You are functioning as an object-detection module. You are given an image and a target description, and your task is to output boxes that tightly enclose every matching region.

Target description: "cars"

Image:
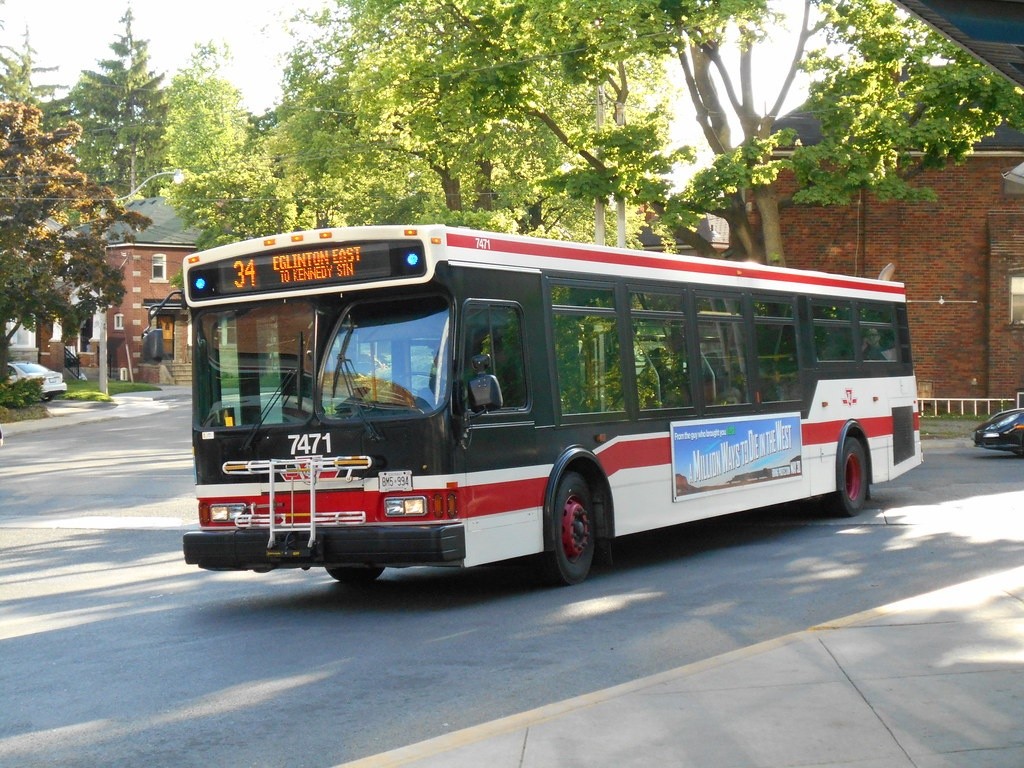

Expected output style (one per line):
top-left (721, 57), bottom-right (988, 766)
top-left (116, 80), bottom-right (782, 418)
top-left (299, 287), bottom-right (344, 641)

top-left (204, 395), bottom-right (313, 426)
top-left (7, 361), bottom-right (67, 401)
top-left (971, 408), bottom-right (1024, 457)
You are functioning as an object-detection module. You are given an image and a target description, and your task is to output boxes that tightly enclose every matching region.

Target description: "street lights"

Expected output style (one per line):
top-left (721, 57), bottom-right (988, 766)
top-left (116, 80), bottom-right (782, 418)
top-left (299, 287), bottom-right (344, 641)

top-left (100, 170), bottom-right (184, 394)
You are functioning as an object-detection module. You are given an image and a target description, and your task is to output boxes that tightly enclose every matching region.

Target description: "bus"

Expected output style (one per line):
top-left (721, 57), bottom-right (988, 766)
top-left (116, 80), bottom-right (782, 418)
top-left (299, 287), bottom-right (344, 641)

top-left (141, 225), bottom-right (924, 585)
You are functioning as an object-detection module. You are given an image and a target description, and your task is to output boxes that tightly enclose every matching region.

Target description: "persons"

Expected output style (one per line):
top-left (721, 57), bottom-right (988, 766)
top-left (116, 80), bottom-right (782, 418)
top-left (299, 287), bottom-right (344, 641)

top-left (862, 329), bottom-right (890, 360)
top-left (639, 349), bottom-right (744, 407)
